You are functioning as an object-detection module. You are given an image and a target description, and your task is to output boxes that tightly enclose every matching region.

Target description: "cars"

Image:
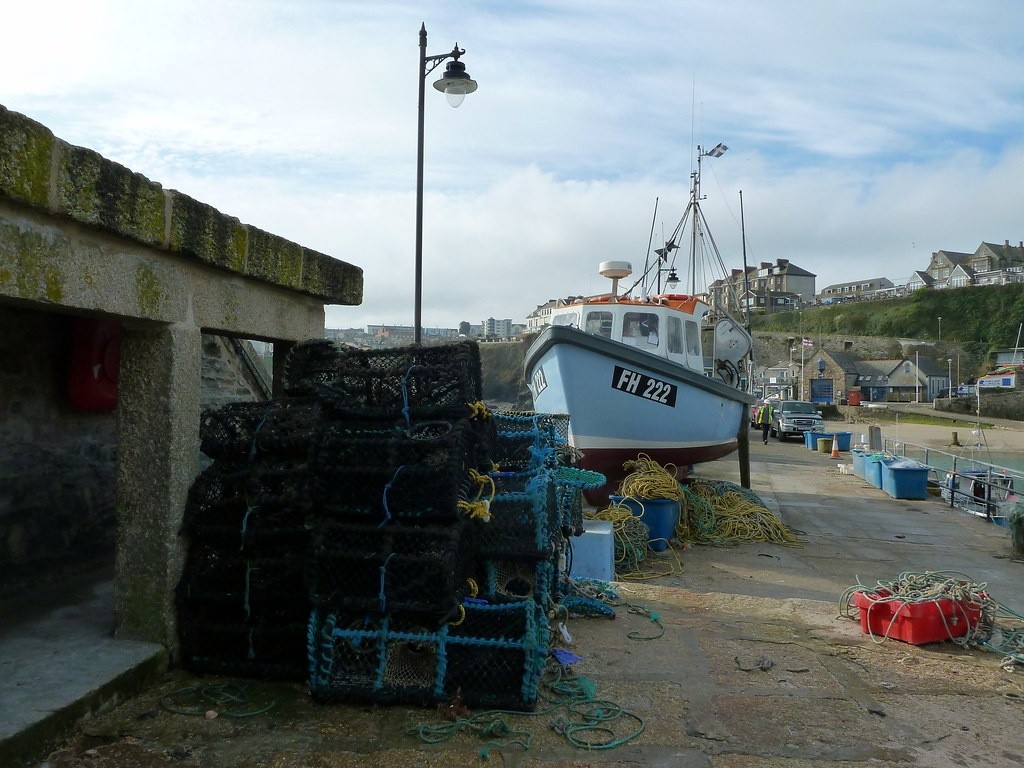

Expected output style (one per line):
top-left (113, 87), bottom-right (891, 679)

top-left (751, 399), bottom-right (781, 430)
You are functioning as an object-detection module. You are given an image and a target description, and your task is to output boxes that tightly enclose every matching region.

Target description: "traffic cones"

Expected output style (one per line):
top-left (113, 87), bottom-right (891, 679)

top-left (828, 433), bottom-right (843, 460)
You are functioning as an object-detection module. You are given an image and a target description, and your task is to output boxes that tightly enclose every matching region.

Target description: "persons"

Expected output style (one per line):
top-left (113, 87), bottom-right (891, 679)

top-left (757, 399), bottom-right (776, 445)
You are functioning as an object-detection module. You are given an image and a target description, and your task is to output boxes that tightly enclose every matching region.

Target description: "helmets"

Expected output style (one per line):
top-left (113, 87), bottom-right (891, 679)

top-left (763, 399), bottom-right (771, 404)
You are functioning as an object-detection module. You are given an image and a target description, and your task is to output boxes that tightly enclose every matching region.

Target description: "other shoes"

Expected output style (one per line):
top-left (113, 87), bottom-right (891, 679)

top-left (765, 440), bottom-right (768, 444)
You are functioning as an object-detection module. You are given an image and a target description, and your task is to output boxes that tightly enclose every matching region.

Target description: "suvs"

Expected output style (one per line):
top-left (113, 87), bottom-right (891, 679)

top-left (769, 400), bottom-right (825, 443)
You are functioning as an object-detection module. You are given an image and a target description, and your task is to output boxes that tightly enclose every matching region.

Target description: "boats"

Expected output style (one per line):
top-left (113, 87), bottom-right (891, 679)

top-left (937, 381), bottom-right (1022, 528)
top-left (519, 72), bottom-right (758, 508)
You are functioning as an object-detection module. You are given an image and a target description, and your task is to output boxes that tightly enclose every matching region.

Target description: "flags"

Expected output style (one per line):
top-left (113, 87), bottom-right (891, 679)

top-left (803, 339), bottom-right (813, 347)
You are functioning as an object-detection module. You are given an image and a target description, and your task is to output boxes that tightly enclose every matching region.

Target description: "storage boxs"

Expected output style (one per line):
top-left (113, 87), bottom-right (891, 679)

top-left (567, 520), bottom-right (615, 584)
top-left (804, 430), bottom-right (851, 452)
top-left (608, 495), bottom-right (678, 551)
top-left (855, 585), bottom-right (990, 647)
top-left (854, 449), bottom-right (933, 499)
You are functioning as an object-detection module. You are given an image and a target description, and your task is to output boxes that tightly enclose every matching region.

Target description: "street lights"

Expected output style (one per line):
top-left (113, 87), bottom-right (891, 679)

top-left (407, 21), bottom-right (480, 386)
top-left (937, 316), bottom-right (943, 342)
top-left (946, 358), bottom-right (955, 404)
top-left (798, 312), bottom-right (803, 333)
